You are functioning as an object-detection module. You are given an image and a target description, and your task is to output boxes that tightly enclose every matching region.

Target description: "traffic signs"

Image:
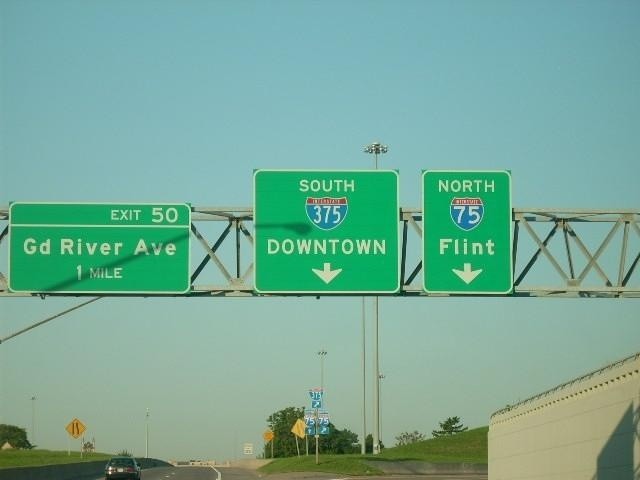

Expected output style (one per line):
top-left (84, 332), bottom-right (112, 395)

top-left (9, 202), bottom-right (189, 293)
top-left (318, 416), bottom-right (330, 426)
top-left (304, 416), bottom-right (315, 426)
top-left (422, 170), bottom-right (512, 293)
top-left (310, 387), bottom-right (325, 400)
top-left (251, 167), bottom-right (401, 293)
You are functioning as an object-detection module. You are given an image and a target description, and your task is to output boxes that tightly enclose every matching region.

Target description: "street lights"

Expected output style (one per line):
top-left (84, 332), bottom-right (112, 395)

top-left (363, 140), bottom-right (391, 454)
top-left (30, 395), bottom-right (37, 450)
top-left (317, 349), bottom-right (328, 386)
top-left (144, 408), bottom-right (152, 457)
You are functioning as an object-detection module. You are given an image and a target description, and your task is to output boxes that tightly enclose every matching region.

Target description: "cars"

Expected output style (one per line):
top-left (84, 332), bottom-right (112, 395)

top-left (103, 454), bottom-right (140, 480)
